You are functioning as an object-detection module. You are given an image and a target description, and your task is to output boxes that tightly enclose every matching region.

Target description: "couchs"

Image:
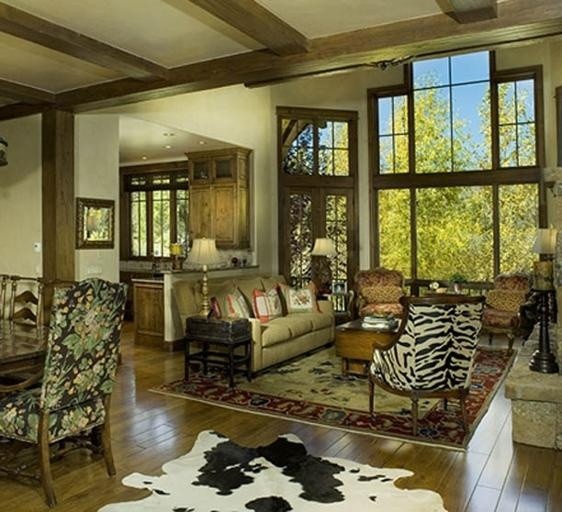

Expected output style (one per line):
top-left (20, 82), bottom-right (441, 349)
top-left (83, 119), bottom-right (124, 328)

top-left (355, 267), bottom-right (407, 318)
top-left (172, 274), bottom-right (335, 372)
top-left (483, 273), bottom-right (530, 351)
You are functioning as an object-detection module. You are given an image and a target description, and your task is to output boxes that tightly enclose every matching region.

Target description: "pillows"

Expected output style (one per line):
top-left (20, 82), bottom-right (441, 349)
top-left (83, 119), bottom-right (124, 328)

top-left (233, 279), bottom-right (263, 317)
top-left (193, 279), bottom-right (234, 311)
top-left (279, 280), bottom-right (320, 313)
top-left (252, 285), bottom-right (282, 323)
top-left (224, 287), bottom-right (250, 318)
top-left (261, 275), bottom-right (287, 313)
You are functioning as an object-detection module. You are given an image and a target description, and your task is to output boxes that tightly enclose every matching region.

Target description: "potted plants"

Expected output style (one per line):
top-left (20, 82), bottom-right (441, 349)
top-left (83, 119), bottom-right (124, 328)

top-left (448, 272), bottom-right (470, 294)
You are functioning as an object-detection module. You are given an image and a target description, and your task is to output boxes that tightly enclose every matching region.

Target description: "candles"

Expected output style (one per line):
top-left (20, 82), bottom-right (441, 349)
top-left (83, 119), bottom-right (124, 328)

top-left (532, 260), bottom-right (553, 290)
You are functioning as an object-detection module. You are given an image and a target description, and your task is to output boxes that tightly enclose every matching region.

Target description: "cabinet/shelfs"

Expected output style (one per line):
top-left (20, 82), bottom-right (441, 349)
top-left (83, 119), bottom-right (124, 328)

top-left (184, 148), bottom-right (253, 249)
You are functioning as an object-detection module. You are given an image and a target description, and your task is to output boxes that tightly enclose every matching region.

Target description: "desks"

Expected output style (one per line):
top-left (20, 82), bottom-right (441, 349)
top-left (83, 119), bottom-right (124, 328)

top-left (0, 317), bottom-right (49, 393)
top-left (335, 316), bottom-right (402, 377)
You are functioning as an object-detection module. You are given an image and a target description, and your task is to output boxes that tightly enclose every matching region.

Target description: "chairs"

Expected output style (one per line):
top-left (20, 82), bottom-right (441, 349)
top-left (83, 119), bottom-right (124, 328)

top-left (52, 278), bottom-right (79, 304)
top-left (369, 296), bottom-right (486, 437)
top-left (0, 274), bottom-right (9, 319)
top-left (0, 278), bottom-right (129, 508)
top-left (9, 277), bottom-right (44, 326)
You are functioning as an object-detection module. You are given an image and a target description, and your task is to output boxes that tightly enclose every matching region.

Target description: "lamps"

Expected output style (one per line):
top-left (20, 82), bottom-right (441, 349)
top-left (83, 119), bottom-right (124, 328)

top-left (531, 229), bottom-right (558, 324)
top-left (310, 238), bottom-right (337, 293)
top-left (169, 243), bottom-right (183, 270)
top-left (183, 237), bottom-right (227, 316)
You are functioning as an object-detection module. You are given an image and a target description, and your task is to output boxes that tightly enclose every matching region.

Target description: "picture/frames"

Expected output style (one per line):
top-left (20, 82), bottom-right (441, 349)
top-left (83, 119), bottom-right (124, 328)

top-left (75, 197), bottom-right (115, 249)
top-left (332, 279), bottom-right (348, 294)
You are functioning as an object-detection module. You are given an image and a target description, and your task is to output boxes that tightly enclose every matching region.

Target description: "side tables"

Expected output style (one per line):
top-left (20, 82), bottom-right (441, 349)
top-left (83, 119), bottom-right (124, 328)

top-left (317, 290), bottom-right (354, 321)
top-left (184, 316), bottom-right (252, 387)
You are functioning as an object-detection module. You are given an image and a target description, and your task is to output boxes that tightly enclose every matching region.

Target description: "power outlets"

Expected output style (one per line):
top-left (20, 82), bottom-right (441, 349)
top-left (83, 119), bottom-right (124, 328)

top-left (33, 242), bottom-right (41, 254)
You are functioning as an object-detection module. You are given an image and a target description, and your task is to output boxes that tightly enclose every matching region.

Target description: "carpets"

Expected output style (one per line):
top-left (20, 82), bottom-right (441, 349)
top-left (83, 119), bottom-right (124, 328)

top-left (147, 342), bottom-right (518, 452)
top-left (97, 430), bottom-right (449, 512)
